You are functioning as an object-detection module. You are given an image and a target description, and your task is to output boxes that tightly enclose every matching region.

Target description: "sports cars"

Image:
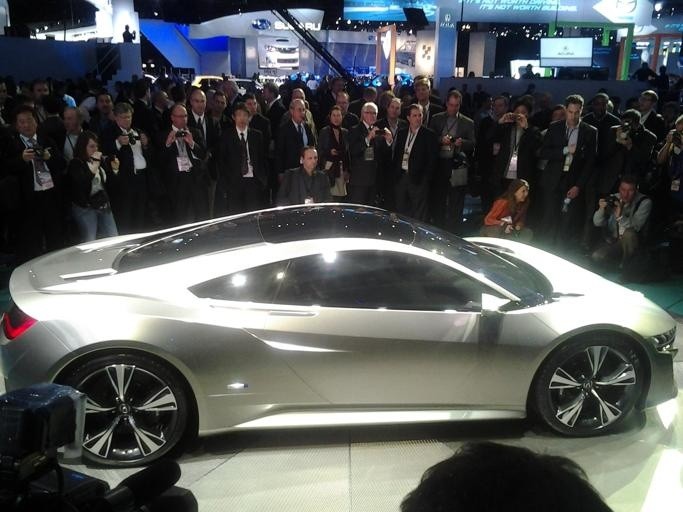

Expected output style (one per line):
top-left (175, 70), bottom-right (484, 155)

top-left (0, 202), bottom-right (679, 467)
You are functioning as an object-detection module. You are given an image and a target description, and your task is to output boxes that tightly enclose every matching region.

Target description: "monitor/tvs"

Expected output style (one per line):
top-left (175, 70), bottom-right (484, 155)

top-left (540, 37), bottom-right (593, 67)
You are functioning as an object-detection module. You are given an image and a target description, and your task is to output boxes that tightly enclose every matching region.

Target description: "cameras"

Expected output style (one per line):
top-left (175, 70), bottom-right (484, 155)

top-left (671, 131), bottom-right (683, 146)
top-left (511, 114), bottom-right (521, 121)
top-left (449, 135), bottom-right (461, 144)
top-left (620, 121), bottom-right (633, 139)
top-left (375, 129), bottom-right (385, 135)
top-left (175, 131), bottom-right (188, 137)
top-left (126, 132), bottom-right (140, 144)
top-left (28, 145), bottom-right (44, 158)
top-left (101, 154), bottom-right (116, 165)
top-left (605, 196), bottom-right (619, 208)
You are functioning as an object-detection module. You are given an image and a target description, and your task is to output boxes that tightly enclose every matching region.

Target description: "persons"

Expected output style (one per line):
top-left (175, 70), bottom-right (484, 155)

top-left (1, 62), bottom-right (683, 275)
top-left (399, 439), bottom-right (617, 511)
top-left (122, 24), bottom-right (135, 43)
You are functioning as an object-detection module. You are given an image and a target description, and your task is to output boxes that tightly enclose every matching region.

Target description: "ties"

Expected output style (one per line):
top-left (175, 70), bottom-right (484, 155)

top-left (240, 133), bottom-right (248, 175)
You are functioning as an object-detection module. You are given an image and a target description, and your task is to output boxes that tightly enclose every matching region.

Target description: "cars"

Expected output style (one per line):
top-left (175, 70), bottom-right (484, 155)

top-left (395, 41), bottom-right (416, 68)
top-left (228, 78), bottom-right (262, 95)
top-left (264, 39), bottom-right (299, 71)
top-left (190, 75), bottom-right (223, 90)
